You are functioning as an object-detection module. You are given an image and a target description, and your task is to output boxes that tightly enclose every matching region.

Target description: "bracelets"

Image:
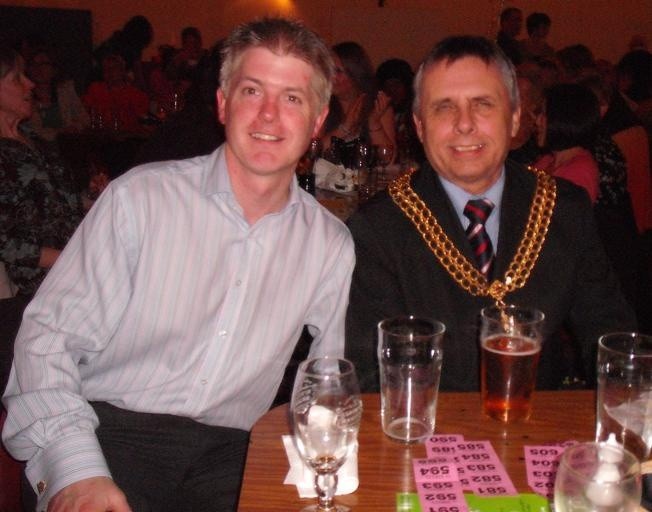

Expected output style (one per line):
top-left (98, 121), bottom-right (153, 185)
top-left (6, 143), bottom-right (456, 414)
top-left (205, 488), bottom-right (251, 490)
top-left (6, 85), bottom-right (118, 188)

top-left (366, 124), bottom-right (386, 132)
top-left (338, 123), bottom-right (352, 136)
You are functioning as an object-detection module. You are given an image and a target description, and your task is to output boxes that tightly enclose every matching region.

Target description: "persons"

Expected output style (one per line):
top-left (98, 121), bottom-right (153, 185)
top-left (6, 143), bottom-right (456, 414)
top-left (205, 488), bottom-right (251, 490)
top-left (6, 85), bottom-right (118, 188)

top-left (24, 45), bottom-right (90, 142)
top-left (527, 82), bottom-right (606, 177)
top-left (535, 62), bottom-right (558, 90)
top-left (85, 54), bottom-right (165, 143)
top-left (168, 26), bottom-right (208, 78)
top-left (558, 45), bottom-right (614, 123)
top-left (0, 48), bottom-right (109, 300)
top-left (85, 15), bottom-right (154, 92)
top-left (0, 8), bottom-right (357, 512)
top-left (523, 13), bottom-right (561, 64)
top-left (284, 35), bottom-right (646, 392)
top-left (136, 55), bottom-right (225, 163)
top-left (293, 44), bottom-right (395, 177)
top-left (154, 62), bottom-right (199, 123)
top-left (602, 50), bottom-right (652, 136)
top-left (510, 75), bottom-right (539, 167)
top-left (493, 7), bottom-right (540, 74)
top-left (369, 57), bottom-right (423, 164)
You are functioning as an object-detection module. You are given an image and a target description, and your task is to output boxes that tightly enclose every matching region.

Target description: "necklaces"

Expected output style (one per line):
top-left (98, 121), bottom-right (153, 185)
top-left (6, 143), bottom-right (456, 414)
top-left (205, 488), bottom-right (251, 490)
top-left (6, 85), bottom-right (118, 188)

top-left (388, 159), bottom-right (557, 337)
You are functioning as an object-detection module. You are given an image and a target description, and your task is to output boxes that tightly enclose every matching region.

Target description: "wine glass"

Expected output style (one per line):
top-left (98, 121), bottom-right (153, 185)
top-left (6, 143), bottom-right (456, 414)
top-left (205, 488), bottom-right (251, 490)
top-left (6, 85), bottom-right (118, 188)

top-left (555, 442), bottom-right (643, 512)
top-left (290, 356), bottom-right (363, 512)
top-left (375, 144), bottom-right (394, 187)
top-left (352, 144), bottom-right (371, 192)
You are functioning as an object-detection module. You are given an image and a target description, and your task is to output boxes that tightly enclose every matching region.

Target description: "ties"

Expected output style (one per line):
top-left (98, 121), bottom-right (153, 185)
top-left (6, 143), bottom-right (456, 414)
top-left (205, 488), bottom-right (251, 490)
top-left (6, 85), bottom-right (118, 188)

top-left (464, 198), bottom-right (495, 280)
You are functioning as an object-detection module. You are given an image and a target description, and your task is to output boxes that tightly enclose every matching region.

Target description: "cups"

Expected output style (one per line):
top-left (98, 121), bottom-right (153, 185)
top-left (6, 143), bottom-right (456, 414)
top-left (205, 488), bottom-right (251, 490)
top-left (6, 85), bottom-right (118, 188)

top-left (596, 333), bottom-right (652, 464)
top-left (481, 305), bottom-right (546, 426)
top-left (300, 139), bottom-right (319, 195)
top-left (379, 315), bottom-right (445, 444)
